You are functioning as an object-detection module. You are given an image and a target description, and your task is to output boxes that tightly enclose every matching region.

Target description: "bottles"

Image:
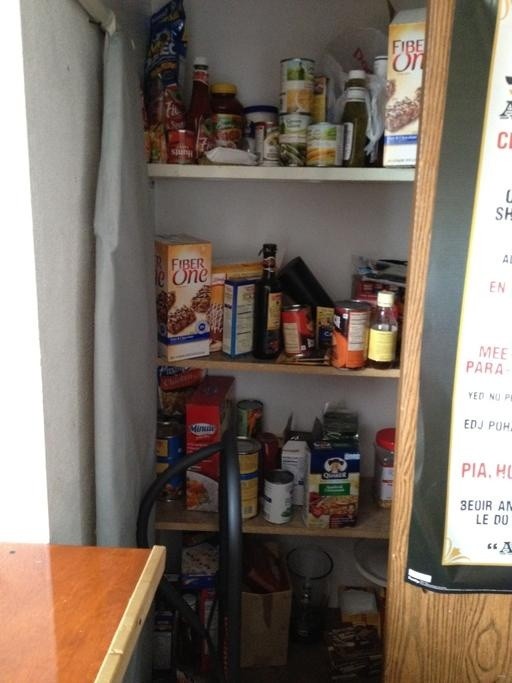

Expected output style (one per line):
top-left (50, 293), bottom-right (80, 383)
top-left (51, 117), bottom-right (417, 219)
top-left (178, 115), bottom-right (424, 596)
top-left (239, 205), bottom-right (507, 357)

top-left (152, 610), bottom-right (174, 676)
top-left (185, 56), bottom-right (212, 162)
top-left (213, 81), bottom-right (245, 157)
top-left (371, 427), bottom-right (396, 510)
top-left (367, 289), bottom-right (403, 368)
top-left (368, 52), bottom-right (387, 165)
top-left (255, 241), bottom-right (282, 358)
top-left (341, 68), bottom-right (370, 165)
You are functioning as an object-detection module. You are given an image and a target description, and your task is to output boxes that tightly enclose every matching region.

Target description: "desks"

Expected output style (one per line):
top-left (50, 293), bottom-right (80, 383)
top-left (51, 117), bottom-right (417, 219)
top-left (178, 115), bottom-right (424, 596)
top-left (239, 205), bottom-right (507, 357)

top-left (1, 540), bottom-right (168, 682)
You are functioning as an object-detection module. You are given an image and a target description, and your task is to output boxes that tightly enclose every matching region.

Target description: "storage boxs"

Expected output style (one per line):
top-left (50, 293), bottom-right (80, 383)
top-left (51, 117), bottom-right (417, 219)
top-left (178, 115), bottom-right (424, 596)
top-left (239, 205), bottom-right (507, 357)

top-left (238, 550), bottom-right (293, 668)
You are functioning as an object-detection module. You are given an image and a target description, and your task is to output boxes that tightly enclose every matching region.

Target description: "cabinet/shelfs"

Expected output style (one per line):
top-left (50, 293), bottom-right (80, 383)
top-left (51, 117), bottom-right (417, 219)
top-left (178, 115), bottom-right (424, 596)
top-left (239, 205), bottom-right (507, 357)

top-left (144, 157), bottom-right (420, 542)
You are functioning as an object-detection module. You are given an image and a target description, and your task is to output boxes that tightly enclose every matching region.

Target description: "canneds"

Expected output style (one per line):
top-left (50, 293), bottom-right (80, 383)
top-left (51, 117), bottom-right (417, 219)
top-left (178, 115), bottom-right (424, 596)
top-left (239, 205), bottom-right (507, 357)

top-left (374, 428), bottom-right (395, 511)
top-left (280, 303), bottom-right (316, 359)
top-left (236, 399), bottom-right (294, 525)
top-left (154, 421), bottom-right (186, 503)
top-left (242, 57), bottom-right (345, 166)
top-left (166, 129), bottom-right (196, 165)
top-left (329, 299), bottom-right (372, 371)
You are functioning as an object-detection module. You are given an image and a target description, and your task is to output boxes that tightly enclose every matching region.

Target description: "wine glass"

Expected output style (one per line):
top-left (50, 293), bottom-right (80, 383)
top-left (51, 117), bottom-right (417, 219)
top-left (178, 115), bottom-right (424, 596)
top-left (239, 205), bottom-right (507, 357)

top-left (285, 545), bottom-right (336, 642)
